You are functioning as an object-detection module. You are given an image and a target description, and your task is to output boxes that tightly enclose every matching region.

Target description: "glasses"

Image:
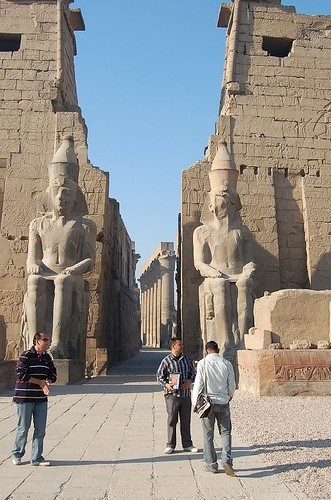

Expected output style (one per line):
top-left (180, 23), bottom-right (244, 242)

top-left (39, 338), bottom-right (49, 342)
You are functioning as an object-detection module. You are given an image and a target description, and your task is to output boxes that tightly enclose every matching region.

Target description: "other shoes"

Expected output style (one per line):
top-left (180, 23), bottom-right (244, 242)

top-left (31, 461), bottom-right (51, 466)
top-left (183, 445), bottom-right (199, 453)
top-left (205, 464), bottom-right (219, 474)
top-left (220, 462), bottom-right (234, 476)
top-left (13, 455), bottom-right (22, 465)
top-left (163, 447), bottom-right (175, 454)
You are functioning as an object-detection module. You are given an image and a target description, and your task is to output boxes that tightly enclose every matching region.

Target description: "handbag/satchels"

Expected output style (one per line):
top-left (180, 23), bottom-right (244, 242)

top-left (193, 393), bottom-right (212, 419)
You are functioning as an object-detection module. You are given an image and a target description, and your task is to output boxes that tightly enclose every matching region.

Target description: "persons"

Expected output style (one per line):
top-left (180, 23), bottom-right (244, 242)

top-left (156, 338), bottom-right (199, 454)
top-left (191, 341), bottom-right (236, 477)
top-left (26, 134), bottom-right (96, 358)
top-left (193, 138), bottom-right (257, 348)
top-left (11, 331), bottom-right (57, 466)
